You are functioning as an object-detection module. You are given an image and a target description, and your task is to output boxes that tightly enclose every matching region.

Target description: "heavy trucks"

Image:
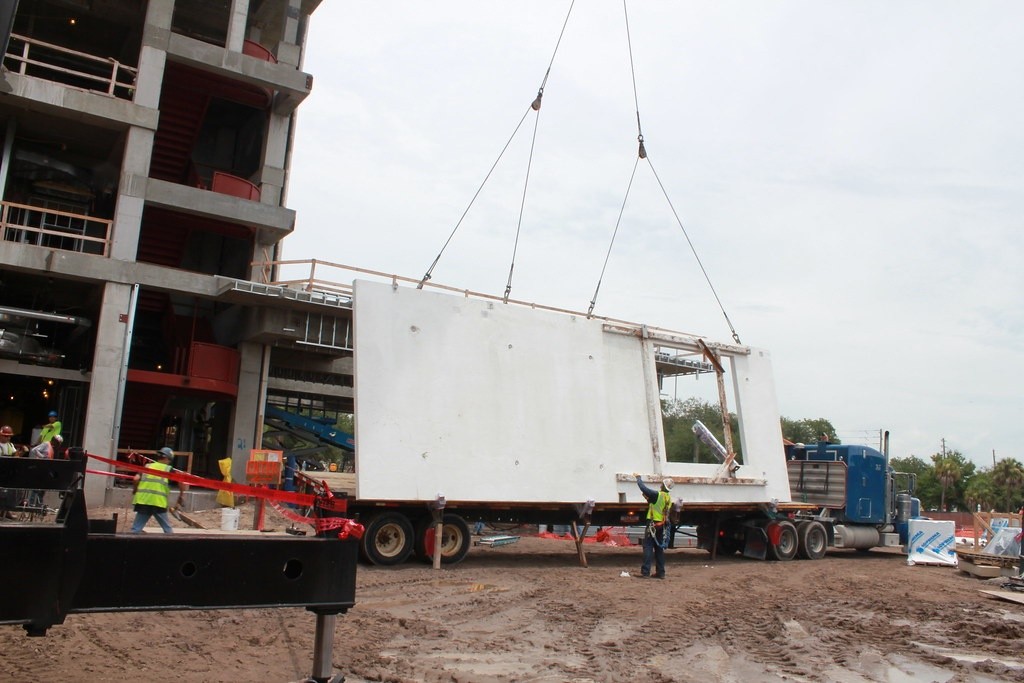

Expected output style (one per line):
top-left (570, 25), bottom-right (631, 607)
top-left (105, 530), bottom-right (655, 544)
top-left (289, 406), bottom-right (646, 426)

top-left (267, 247), bottom-right (930, 569)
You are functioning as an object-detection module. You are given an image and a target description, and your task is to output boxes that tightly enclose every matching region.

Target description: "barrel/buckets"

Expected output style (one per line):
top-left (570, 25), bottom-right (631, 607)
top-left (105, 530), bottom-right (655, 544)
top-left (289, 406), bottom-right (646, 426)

top-left (219, 507), bottom-right (241, 531)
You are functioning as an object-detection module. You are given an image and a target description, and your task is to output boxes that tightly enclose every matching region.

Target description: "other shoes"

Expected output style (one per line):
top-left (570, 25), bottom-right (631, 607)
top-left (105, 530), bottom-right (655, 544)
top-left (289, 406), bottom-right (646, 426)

top-left (651, 574), bottom-right (666, 579)
top-left (633, 574), bottom-right (650, 578)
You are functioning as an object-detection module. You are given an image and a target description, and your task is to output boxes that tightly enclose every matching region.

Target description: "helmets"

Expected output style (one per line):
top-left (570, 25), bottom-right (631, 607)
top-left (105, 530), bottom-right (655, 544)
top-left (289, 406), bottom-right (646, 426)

top-left (0, 425), bottom-right (14, 435)
top-left (663, 478), bottom-right (674, 491)
top-left (48, 410), bottom-right (58, 416)
top-left (157, 446), bottom-right (174, 461)
top-left (53, 435), bottom-right (63, 444)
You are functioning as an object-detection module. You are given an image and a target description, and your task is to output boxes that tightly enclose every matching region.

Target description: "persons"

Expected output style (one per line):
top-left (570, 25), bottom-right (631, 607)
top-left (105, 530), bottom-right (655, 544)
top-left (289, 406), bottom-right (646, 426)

top-left (0, 426), bottom-right (27, 519)
top-left (635, 473), bottom-right (675, 579)
top-left (27, 434), bottom-right (64, 515)
top-left (38, 412), bottom-right (61, 444)
top-left (472, 521), bottom-right (485, 536)
top-left (130, 447), bottom-right (175, 533)
